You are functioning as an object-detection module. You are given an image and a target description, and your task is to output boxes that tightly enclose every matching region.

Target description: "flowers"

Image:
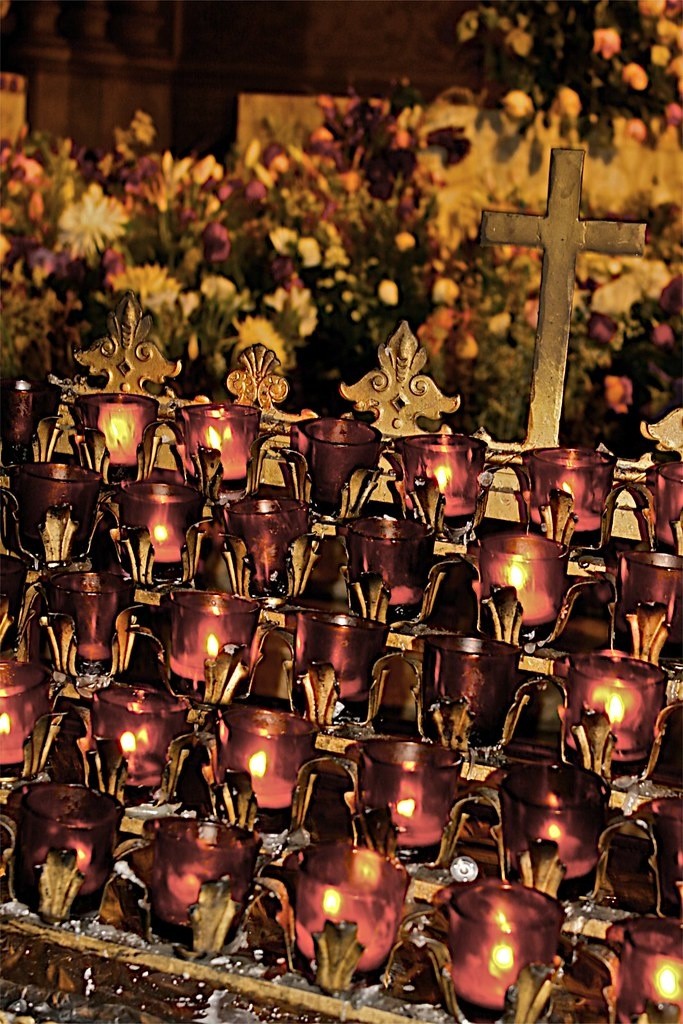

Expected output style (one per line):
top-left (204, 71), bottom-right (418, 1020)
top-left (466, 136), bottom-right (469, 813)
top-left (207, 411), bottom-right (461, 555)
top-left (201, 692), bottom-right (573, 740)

top-left (0, 0), bottom-right (683, 461)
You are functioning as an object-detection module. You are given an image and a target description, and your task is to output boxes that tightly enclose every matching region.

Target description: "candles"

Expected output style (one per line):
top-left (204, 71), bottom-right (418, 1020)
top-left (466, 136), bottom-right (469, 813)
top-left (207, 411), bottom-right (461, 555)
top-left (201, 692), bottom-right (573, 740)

top-left (604, 918), bottom-right (683, 1024)
top-left (639, 797), bottom-right (683, 918)
top-left (5, 782), bottom-right (125, 917)
top-left (88, 685), bottom-right (190, 795)
top-left (0, 376), bottom-right (683, 626)
top-left (430, 883), bottom-right (565, 1010)
top-left (346, 737), bottom-right (461, 849)
top-left (423, 633), bottom-right (522, 746)
top-left (284, 847), bottom-right (411, 981)
top-left (141, 818), bottom-right (264, 947)
top-left (41, 571), bottom-right (135, 672)
top-left (156, 588), bottom-right (260, 691)
top-left (219, 703), bottom-right (318, 810)
top-left (552, 653), bottom-right (668, 775)
top-left (0, 660), bottom-right (52, 774)
top-left (485, 761), bottom-right (613, 888)
top-left (292, 609), bottom-right (389, 718)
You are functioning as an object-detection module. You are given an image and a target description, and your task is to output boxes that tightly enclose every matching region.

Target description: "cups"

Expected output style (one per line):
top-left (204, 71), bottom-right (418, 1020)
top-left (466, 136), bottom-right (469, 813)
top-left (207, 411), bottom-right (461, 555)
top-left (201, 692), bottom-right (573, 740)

top-left (0, 381), bottom-right (683, 1024)
